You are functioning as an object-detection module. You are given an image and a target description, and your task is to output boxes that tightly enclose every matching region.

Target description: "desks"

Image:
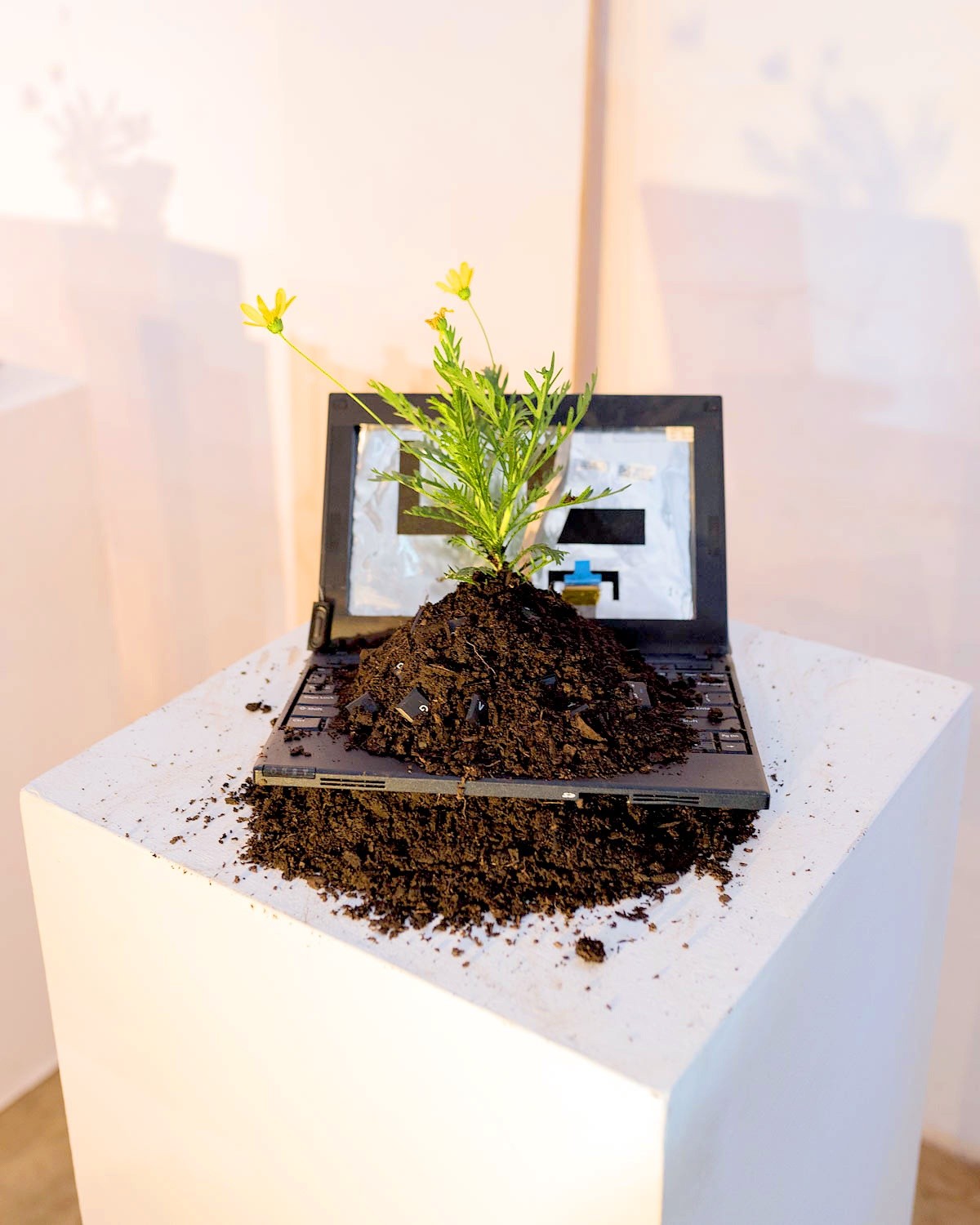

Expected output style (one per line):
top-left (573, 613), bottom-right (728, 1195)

top-left (11, 619), bottom-right (976, 1225)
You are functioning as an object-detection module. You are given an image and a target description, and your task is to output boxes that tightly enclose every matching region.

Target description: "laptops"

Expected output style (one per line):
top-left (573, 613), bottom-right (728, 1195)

top-left (255, 391), bottom-right (771, 812)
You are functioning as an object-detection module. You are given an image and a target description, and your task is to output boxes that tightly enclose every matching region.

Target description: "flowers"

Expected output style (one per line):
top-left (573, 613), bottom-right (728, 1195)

top-left (234, 254), bottom-right (637, 590)
